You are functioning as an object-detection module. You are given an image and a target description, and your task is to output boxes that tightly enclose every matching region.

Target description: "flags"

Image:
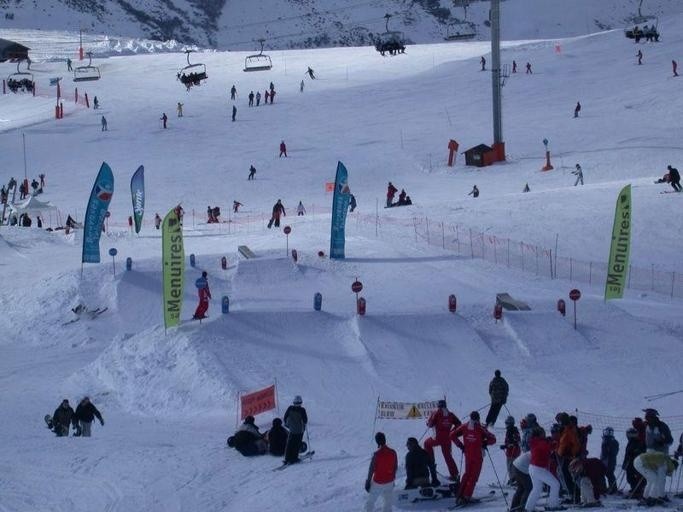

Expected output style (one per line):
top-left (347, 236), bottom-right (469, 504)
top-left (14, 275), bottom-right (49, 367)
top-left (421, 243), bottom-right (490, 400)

top-left (132, 166), bottom-right (144, 232)
top-left (330, 162), bottom-right (348, 258)
top-left (83, 161), bottom-right (115, 262)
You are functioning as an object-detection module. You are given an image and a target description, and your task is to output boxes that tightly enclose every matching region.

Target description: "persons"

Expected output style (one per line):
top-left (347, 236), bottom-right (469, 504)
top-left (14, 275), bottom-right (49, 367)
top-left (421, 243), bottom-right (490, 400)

top-left (160, 72), bottom-right (200, 128)
top-left (485, 370), bottom-right (509, 427)
top-left (8, 78), bottom-right (33, 92)
top-left (449, 411), bottom-right (497, 505)
top-left (386, 181), bottom-right (411, 207)
top-left (75, 396), bottom-right (104, 437)
top-left (66, 58), bottom-right (72, 71)
top-left (279, 140), bottom-right (287, 157)
top-left (193, 271), bottom-right (212, 318)
top-left (129, 213), bottom-right (162, 229)
top-left (405, 400), bottom-right (462, 490)
top-left (364, 432), bottom-right (398, 511)
top-left (349, 194), bottom-right (356, 211)
top-left (623, 409), bottom-right (683, 506)
top-left (227, 396), bottom-right (308, 464)
top-left (53, 399), bottom-right (75, 437)
top-left (2, 174), bottom-right (76, 231)
top-left (94, 96), bottom-right (107, 130)
top-left (248, 164), bottom-right (255, 179)
top-left (207, 201), bottom-right (243, 223)
top-left (267, 199), bottom-right (305, 228)
top-left (231, 66), bottom-right (315, 121)
top-left (500, 412), bottom-right (619, 512)
top-left (468, 24), bottom-right (683, 197)
top-left (378, 38), bottom-right (405, 56)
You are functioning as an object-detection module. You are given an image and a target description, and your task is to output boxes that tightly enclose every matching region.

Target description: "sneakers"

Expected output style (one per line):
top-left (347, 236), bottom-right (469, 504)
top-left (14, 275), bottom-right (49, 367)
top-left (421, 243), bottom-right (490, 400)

top-left (582, 501), bottom-right (601, 508)
top-left (544, 497), bottom-right (601, 511)
top-left (607, 487), bottom-right (640, 499)
top-left (456, 496), bottom-right (476, 504)
top-left (640, 496), bottom-right (670, 506)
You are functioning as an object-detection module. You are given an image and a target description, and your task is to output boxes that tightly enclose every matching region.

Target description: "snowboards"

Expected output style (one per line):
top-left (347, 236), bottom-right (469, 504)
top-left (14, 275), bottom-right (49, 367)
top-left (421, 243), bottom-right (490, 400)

top-left (393, 486), bottom-right (435, 501)
top-left (45, 414), bottom-right (69, 437)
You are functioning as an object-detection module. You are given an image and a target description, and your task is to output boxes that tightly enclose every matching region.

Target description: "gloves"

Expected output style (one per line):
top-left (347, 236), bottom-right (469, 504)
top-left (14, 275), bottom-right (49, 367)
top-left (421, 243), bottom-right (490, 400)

top-left (500, 445), bottom-right (506, 449)
top-left (365, 480), bottom-right (371, 492)
top-left (460, 446), bottom-right (464, 451)
top-left (482, 444), bottom-right (488, 449)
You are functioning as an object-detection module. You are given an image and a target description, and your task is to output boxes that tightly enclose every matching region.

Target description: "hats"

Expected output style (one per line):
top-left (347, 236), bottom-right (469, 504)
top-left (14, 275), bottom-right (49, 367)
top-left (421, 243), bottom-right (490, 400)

top-left (439, 400), bottom-right (446, 407)
top-left (407, 438), bottom-right (418, 446)
top-left (293, 395), bottom-right (302, 404)
top-left (603, 427), bottom-right (614, 437)
top-left (202, 272), bottom-right (207, 277)
top-left (246, 416), bottom-right (254, 423)
top-left (273, 418), bottom-right (282, 426)
top-left (375, 432), bottom-right (385, 444)
top-left (505, 414), bottom-right (536, 429)
top-left (471, 411), bottom-right (480, 420)
top-left (551, 423), bottom-right (562, 433)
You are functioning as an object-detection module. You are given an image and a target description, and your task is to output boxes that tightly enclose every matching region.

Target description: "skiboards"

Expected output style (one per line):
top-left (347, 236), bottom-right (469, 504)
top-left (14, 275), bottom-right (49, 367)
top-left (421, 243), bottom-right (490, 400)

top-left (438, 491), bottom-right (508, 512)
top-left (272, 449), bottom-right (315, 473)
